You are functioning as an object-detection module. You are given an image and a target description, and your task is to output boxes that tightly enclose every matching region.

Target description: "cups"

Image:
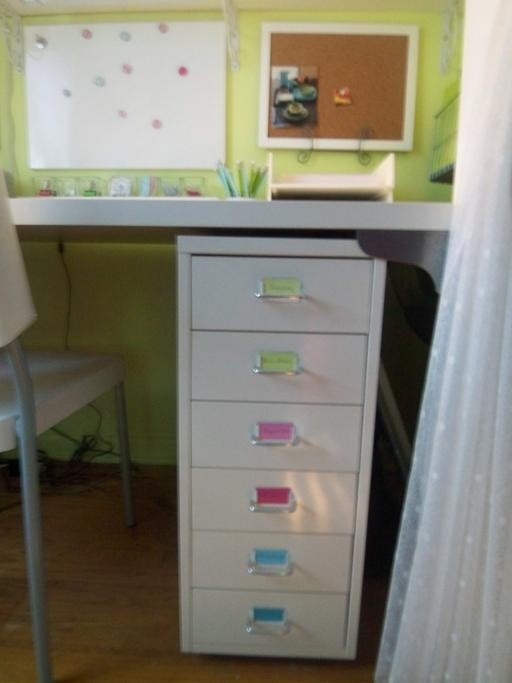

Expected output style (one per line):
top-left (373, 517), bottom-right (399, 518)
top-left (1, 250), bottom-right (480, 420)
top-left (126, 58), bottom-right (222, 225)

top-left (299, 86), bottom-right (315, 97)
top-left (287, 103), bottom-right (304, 116)
top-left (31, 174), bottom-right (205, 197)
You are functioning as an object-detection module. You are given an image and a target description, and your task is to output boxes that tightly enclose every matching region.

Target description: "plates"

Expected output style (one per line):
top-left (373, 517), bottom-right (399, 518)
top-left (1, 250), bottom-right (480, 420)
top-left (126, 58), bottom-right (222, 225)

top-left (293, 87), bottom-right (318, 101)
top-left (281, 107), bottom-right (309, 122)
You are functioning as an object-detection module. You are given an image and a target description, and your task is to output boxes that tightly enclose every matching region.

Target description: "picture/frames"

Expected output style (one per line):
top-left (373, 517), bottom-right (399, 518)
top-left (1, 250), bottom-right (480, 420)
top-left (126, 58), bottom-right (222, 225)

top-left (258, 21), bottom-right (419, 150)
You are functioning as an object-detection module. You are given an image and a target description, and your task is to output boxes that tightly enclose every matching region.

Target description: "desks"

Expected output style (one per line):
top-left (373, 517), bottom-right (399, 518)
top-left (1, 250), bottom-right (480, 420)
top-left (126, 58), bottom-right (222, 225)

top-left (9, 194), bottom-right (454, 475)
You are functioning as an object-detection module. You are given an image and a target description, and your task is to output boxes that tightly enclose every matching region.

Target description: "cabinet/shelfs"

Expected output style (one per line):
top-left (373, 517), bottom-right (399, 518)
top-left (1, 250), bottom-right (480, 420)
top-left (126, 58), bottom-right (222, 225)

top-left (175, 236), bottom-right (395, 664)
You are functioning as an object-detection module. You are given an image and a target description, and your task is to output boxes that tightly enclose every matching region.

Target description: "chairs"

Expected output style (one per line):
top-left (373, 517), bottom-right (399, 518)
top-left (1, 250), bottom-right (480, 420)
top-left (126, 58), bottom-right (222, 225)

top-left (0, 164), bottom-right (150, 683)
